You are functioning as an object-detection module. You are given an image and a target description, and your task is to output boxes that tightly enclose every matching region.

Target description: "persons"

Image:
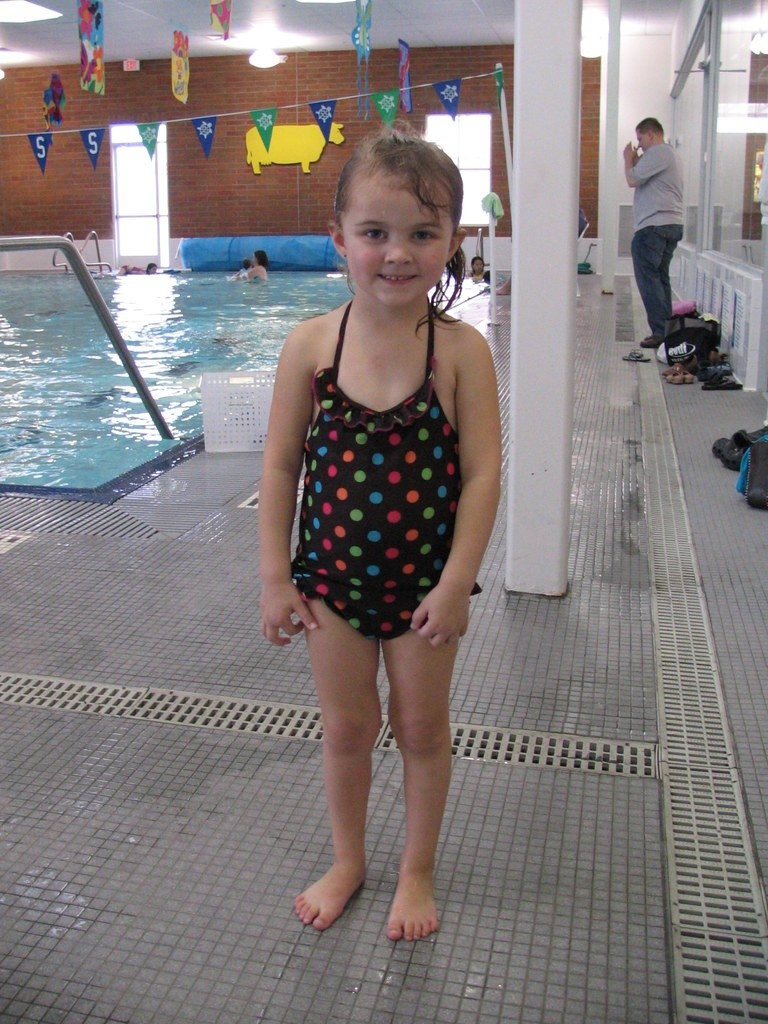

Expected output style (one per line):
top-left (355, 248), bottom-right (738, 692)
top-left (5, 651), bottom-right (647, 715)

top-left (465, 257), bottom-right (511, 296)
top-left (260, 120), bottom-right (501, 939)
top-left (622, 118), bottom-right (686, 352)
top-left (117, 262), bottom-right (158, 277)
top-left (231, 251), bottom-right (269, 285)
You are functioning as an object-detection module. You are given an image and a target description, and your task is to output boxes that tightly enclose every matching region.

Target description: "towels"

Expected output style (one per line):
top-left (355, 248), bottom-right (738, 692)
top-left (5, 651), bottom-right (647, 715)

top-left (481, 192), bottom-right (504, 220)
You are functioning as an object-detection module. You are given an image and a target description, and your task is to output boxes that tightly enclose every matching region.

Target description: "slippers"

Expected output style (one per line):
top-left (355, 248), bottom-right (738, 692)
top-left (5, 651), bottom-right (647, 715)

top-left (622, 349), bottom-right (651, 362)
top-left (665, 371), bottom-right (695, 385)
top-left (700, 373), bottom-right (743, 390)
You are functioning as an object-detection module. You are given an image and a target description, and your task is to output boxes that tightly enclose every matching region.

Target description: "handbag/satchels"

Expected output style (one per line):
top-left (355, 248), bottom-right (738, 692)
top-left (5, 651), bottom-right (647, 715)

top-left (664, 314), bottom-right (719, 365)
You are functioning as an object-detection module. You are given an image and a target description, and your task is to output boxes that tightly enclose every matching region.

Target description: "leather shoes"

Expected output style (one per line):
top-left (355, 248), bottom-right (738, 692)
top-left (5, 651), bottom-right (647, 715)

top-left (640, 336), bottom-right (665, 348)
